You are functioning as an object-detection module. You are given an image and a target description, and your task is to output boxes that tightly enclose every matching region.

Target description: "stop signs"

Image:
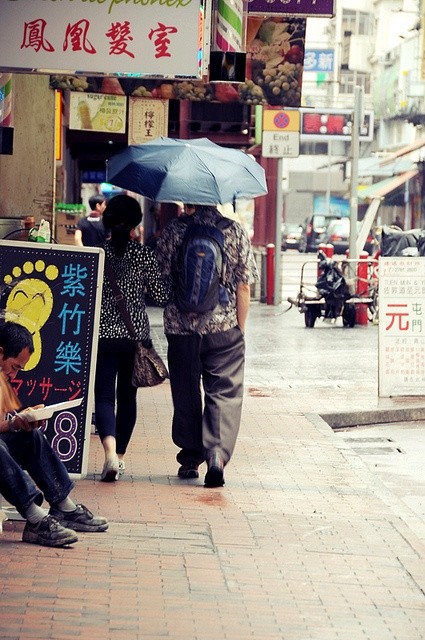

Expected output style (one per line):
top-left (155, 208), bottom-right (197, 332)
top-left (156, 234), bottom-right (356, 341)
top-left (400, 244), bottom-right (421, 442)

top-left (261, 108), bottom-right (301, 158)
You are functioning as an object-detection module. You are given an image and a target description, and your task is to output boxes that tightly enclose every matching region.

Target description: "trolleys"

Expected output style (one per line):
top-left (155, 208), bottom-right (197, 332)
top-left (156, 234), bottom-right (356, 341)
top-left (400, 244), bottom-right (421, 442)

top-left (287, 259), bottom-right (379, 328)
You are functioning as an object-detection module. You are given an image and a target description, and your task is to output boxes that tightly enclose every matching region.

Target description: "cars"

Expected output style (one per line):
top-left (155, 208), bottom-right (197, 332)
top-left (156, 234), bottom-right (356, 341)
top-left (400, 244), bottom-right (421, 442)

top-left (324, 219), bottom-right (374, 256)
top-left (281, 223), bottom-right (303, 251)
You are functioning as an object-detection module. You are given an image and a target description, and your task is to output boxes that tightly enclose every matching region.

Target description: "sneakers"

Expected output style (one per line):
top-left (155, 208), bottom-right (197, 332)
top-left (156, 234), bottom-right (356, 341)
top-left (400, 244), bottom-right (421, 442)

top-left (100, 457), bottom-right (120, 482)
top-left (47, 503), bottom-right (108, 532)
top-left (204, 453), bottom-right (224, 488)
top-left (118, 460), bottom-right (126, 472)
top-left (178, 465), bottom-right (200, 479)
top-left (22, 514), bottom-right (78, 547)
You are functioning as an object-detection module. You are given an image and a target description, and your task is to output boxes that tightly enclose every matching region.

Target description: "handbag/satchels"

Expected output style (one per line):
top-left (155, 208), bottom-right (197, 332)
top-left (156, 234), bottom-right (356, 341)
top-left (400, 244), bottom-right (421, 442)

top-left (131, 343), bottom-right (169, 388)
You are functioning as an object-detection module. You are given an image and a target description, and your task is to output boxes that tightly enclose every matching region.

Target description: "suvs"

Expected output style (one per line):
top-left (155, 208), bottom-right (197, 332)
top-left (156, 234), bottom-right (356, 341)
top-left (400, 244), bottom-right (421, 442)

top-left (299, 214), bottom-right (329, 253)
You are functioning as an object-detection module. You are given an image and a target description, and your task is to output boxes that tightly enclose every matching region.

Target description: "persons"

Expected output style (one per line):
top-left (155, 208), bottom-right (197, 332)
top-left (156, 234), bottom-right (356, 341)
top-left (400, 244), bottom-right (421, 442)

top-left (391, 216), bottom-right (404, 230)
top-left (0, 321), bottom-right (109, 546)
top-left (149, 202), bottom-right (258, 488)
top-left (95, 195), bottom-right (173, 481)
top-left (75, 195), bottom-right (108, 248)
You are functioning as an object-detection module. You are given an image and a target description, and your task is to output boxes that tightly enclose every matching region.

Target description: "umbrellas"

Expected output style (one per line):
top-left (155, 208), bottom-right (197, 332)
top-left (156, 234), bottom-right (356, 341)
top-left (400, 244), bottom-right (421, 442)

top-left (104, 138), bottom-right (270, 234)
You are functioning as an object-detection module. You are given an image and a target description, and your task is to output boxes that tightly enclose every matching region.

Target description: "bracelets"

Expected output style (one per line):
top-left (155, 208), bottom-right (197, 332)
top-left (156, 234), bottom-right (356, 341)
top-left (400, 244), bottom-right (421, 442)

top-left (8, 414), bottom-right (22, 433)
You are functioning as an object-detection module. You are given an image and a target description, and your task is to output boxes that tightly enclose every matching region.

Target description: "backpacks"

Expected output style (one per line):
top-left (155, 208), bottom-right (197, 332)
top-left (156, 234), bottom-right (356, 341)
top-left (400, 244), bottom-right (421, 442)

top-left (169, 214), bottom-right (234, 315)
